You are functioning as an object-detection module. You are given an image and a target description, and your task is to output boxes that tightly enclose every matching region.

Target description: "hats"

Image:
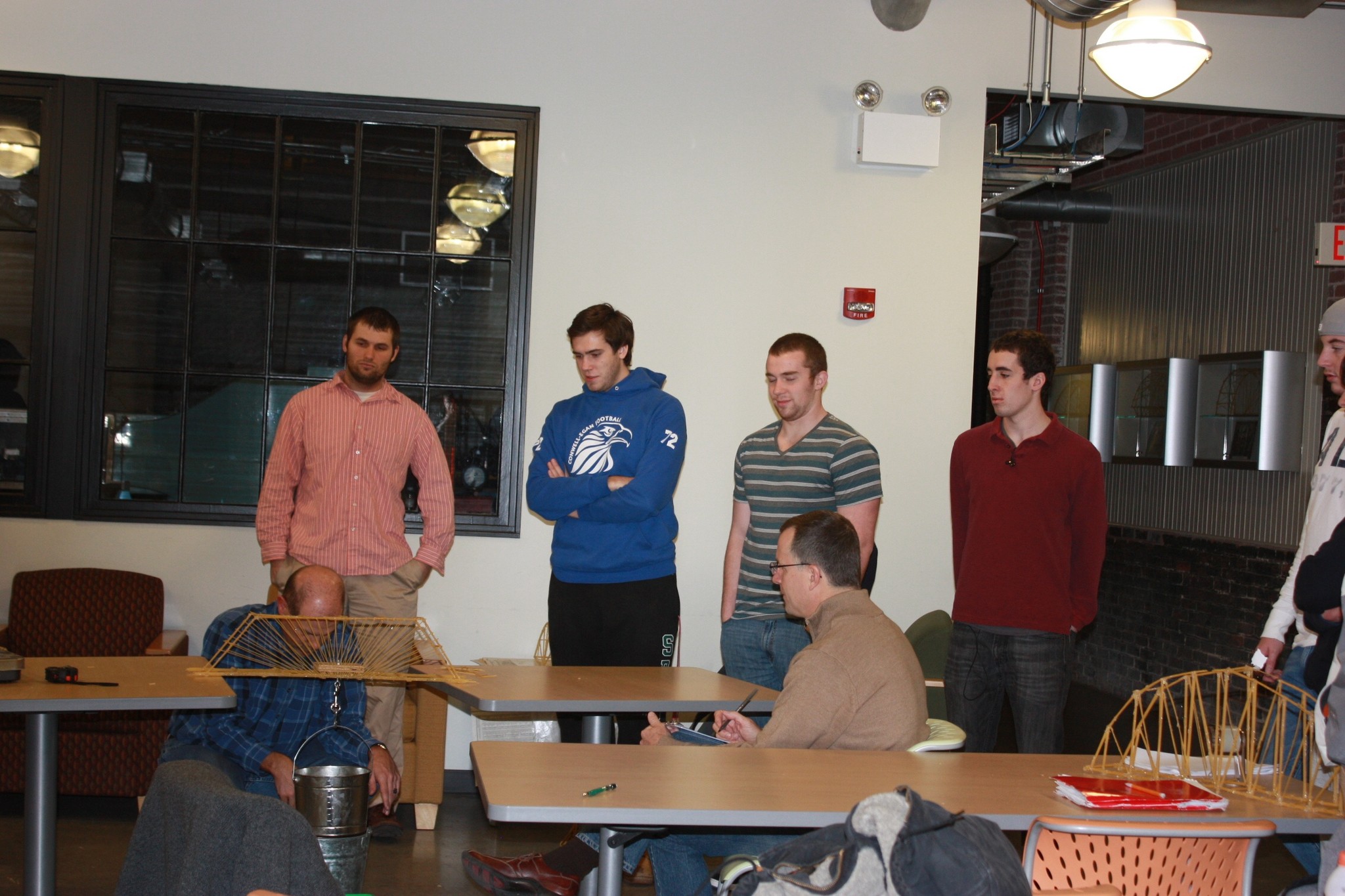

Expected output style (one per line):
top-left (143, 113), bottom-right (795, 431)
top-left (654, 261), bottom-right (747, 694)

top-left (1319, 296), bottom-right (1345, 336)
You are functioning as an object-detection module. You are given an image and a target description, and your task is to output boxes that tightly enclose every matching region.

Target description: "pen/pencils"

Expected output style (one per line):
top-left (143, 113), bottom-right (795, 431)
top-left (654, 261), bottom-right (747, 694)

top-left (583, 783), bottom-right (617, 796)
top-left (1126, 782), bottom-right (1166, 798)
top-left (718, 688), bottom-right (759, 732)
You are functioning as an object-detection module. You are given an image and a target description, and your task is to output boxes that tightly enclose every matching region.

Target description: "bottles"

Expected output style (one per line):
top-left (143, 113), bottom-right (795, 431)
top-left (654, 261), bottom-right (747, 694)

top-left (1324, 851), bottom-right (1345, 896)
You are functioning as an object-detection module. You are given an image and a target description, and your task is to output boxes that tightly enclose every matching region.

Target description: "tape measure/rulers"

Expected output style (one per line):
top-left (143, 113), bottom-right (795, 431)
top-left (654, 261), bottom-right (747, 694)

top-left (45, 665), bottom-right (78, 684)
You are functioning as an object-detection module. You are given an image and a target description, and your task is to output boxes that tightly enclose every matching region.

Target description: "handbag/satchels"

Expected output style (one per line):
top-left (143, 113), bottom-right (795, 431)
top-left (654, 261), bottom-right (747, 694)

top-left (713, 782), bottom-right (1031, 896)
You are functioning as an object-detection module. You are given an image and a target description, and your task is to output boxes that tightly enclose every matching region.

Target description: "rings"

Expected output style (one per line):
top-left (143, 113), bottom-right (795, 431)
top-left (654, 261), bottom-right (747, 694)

top-left (393, 789), bottom-right (397, 794)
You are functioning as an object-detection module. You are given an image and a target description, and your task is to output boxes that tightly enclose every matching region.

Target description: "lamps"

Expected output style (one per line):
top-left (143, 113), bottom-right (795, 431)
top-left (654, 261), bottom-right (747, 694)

top-left (435, 130), bottom-right (515, 263)
top-left (1087, 0), bottom-right (1213, 99)
top-left (853, 79), bottom-right (953, 168)
top-left (0, 119), bottom-right (41, 178)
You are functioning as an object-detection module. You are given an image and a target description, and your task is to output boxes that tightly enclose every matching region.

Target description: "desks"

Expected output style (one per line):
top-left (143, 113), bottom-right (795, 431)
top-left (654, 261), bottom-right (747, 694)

top-left (406, 664), bottom-right (783, 745)
top-left (468, 738), bottom-right (1344, 895)
top-left (0, 655), bottom-right (236, 896)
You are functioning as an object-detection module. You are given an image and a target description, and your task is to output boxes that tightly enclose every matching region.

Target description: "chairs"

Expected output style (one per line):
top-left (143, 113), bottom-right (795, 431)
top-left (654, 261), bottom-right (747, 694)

top-left (716, 717), bottom-right (966, 895)
top-left (1022, 813), bottom-right (1277, 894)
top-left (0, 565), bottom-right (189, 813)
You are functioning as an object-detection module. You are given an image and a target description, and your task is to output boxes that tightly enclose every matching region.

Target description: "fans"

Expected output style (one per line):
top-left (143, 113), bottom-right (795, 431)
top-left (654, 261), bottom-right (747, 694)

top-left (868, 0), bottom-right (1327, 106)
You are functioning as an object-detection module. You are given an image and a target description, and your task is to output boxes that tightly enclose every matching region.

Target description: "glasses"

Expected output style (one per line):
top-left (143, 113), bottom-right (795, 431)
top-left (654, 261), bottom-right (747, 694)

top-left (768, 561), bottom-right (812, 574)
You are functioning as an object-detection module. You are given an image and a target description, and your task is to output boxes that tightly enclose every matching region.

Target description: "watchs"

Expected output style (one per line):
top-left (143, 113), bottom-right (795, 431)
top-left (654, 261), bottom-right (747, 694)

top-left (376, 743), bottom-right (386, 749)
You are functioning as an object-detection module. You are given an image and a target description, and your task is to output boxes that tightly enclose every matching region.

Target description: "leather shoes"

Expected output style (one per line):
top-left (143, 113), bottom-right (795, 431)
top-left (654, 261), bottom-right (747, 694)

top-left (370, 809), bottom-right (401, 839)
top-left (460, 849), bottom-right (582, 893)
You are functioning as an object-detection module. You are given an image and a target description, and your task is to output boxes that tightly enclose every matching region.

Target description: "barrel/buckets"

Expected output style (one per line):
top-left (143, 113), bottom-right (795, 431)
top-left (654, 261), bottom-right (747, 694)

top-left (292, 724), bottom-right (374, 837)
top-left (315, 828), bottom-right (372, 894)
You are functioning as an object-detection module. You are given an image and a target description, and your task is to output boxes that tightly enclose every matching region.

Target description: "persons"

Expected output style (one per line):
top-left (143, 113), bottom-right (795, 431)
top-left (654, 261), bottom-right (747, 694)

top-left (157, 565), bottom-right (403, 817)
top-left (949, 332), bottom-right (1109, 754)
top-left (526, 305), bottom-right (687, 886)
top-left (256, 305), bottom-right (457, 840)
top-left (0, 339), bottom-right (28, 483)
top-left (435, 391), bottom-right (488, 497)
top-left (461, 513), bottom-right (929, 896)
top-left (719, 333), bottom-right (883, 729)
top-left (1250, 297), bottom-right (1345, 896)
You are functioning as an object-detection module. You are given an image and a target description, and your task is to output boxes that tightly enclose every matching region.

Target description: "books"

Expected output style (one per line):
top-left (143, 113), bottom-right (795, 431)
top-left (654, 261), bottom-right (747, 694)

top-left (1052, 772), bottom-right (1227, 808)
